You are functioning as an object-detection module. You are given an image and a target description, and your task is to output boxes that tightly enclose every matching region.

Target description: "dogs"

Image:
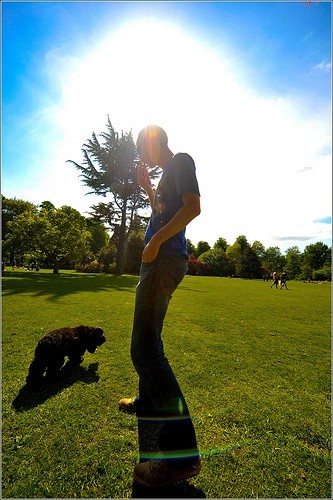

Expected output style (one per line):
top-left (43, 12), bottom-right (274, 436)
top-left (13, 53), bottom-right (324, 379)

top-left (26, 325), bottom-right (105, 380)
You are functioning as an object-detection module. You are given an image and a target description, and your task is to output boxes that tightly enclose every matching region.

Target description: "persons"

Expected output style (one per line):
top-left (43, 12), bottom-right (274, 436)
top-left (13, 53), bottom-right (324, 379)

top-left (116, 125), bottom-right (203, 483)
top-left (280, 270), bottom-right (289, 290)
top-left (271, 270), bottom-right (278, 289)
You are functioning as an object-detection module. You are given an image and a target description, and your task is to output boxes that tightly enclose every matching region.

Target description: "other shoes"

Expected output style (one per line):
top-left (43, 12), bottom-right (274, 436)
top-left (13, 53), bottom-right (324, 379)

top-left (119, 396), bottom-right (137, 410)
top-left (133, 452), bottom-right (201, 487)
